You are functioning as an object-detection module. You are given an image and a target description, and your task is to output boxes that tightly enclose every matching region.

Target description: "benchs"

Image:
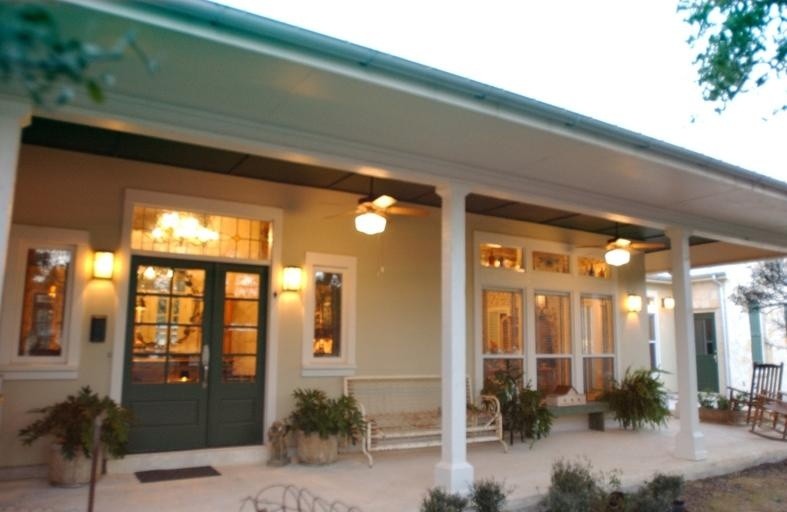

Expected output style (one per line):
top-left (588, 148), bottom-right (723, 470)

top-left (342, 372), bottom-right (510, 469)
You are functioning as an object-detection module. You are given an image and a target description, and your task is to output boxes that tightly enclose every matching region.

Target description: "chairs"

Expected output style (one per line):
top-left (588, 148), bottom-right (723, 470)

top-left (725, 361), bottom-right (786, 441)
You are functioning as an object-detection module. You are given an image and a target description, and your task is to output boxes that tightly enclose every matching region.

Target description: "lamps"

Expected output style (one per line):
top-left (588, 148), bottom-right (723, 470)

top-left (353, 207), bottom-right (388, 237)
top-left (603, 247), bottom-right (630, 267)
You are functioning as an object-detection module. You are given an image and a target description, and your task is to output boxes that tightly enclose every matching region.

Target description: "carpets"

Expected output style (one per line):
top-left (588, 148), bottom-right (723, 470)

top-left (132, 465), bottom-right (222, 483)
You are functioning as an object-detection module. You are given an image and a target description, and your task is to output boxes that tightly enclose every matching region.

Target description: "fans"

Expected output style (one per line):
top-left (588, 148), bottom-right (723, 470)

top-left (317, 177), bottom-right (430, 221)
top-left (575, 222), bottom-right (665, 256)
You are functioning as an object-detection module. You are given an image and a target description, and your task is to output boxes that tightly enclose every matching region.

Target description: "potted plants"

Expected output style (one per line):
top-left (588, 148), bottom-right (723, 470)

top-left (697, 386), bottom-right (732, 424)
top-left (280, 387), bottom-right (376, 465)
top-left (13, 382), bottom-right (140, 488)
